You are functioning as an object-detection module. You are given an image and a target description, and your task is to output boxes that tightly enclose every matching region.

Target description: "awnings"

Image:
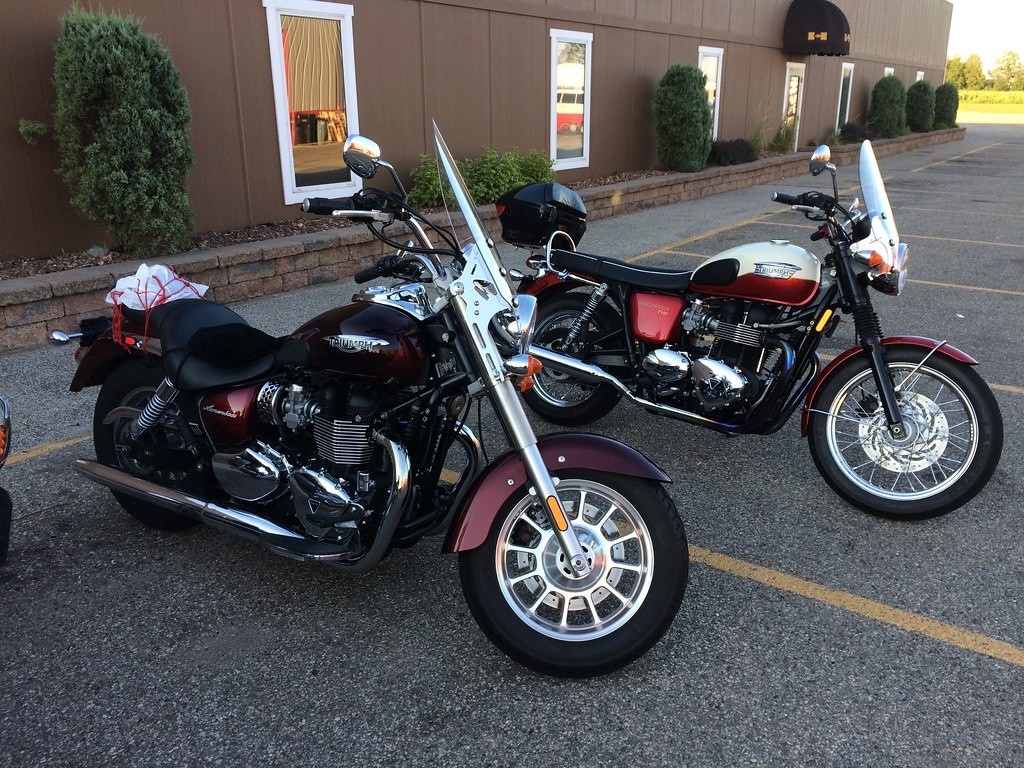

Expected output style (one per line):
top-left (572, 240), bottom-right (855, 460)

top-left (782, 0), bottom-right (850, 56)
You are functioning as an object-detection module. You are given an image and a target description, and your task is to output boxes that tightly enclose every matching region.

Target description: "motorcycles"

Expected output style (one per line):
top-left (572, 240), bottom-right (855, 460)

top-left (48, 118), bottom-right (691, 682)
top-left (493, 139), bottom-right (1005, 521)
